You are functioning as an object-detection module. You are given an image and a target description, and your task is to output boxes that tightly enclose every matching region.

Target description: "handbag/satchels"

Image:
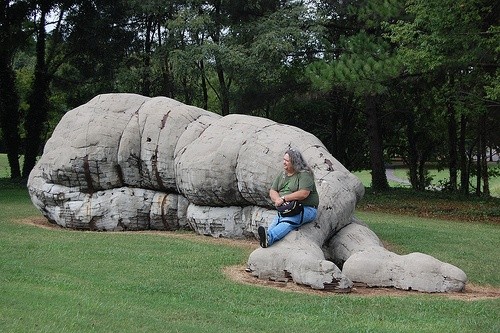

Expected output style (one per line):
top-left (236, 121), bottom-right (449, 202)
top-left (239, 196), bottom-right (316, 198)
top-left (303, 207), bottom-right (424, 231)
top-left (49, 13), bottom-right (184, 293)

top-left (276, 197), bottom-right (304, 225)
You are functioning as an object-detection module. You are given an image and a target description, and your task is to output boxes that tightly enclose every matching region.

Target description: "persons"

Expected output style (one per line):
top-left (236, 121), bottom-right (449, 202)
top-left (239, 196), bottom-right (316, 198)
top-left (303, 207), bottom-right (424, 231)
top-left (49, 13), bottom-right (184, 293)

top-left (257, 148), bottom-right (319, 248)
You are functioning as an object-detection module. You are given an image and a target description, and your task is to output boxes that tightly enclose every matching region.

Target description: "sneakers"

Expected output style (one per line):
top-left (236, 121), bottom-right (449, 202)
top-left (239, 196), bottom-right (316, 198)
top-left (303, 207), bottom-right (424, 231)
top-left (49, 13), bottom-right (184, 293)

top-left (257, 225), bottom-right (269, 248)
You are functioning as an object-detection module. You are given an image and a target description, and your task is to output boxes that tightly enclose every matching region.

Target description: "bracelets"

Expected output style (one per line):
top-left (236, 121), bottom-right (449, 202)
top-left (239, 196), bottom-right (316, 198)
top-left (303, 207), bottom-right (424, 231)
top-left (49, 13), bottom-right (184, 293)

top-left (280, 196), bottom-right (286, 202)
